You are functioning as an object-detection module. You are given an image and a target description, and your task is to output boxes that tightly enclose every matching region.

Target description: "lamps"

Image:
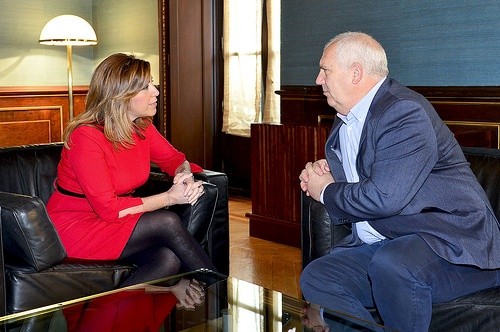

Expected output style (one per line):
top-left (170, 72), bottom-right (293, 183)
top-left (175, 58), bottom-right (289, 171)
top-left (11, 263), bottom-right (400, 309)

top-left (39, 14), bottom-right (98, 122)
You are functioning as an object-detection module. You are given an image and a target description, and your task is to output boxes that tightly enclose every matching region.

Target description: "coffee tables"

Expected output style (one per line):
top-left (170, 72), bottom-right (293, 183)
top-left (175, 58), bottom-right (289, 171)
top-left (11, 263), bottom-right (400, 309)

top-left (0, 268), bottom-right (395, 332)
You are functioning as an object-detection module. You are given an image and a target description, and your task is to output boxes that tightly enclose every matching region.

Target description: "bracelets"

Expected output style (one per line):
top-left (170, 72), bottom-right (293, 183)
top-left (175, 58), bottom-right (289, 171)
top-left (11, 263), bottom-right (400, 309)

top-left (183, 170), bottom-right (194, 179)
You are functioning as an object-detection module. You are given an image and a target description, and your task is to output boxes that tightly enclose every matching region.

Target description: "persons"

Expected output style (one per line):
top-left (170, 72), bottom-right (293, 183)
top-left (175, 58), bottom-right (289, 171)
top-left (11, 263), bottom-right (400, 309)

top-left (46, 53), bottom-right (219, 289)
top-left (301, 304), bottom-right (388, 332)
top-left (299, 31), bottom-right (499, 332)
top-left (63, 278), bottom-right (206, 332)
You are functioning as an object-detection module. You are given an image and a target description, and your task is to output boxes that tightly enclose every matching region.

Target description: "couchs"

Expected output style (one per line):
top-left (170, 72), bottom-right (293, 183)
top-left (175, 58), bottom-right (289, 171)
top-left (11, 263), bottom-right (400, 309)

top-left (299, 145), bottom-right (500, 332)
top-left (0, 142), bottom-right (229, 315)
